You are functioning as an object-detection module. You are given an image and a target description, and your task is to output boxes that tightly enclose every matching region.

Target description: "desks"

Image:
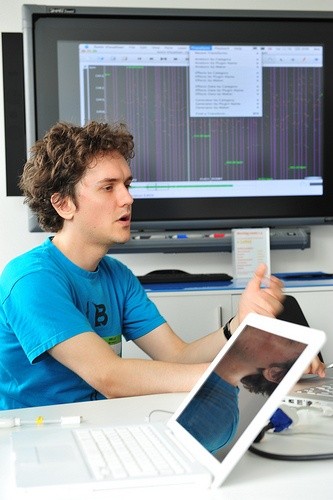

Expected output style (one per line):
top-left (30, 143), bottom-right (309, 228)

top-left (0, 373), bottom-right (333, 499)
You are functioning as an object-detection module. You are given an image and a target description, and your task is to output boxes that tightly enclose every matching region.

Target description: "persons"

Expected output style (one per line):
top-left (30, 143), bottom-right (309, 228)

top-left (177, 325), bottom-right (310, 456)
top-left (0, 122), bottom-right (327, 412)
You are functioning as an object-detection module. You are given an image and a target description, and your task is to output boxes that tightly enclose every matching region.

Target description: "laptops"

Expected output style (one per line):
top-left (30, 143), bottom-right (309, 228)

top-left (0, 310), bottom-right (327, 500)
top-left (281, 368), bottom-right (333, 411)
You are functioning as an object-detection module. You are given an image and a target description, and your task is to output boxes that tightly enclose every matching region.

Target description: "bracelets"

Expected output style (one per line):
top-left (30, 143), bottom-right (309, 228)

top-left (222, 315), bottom-right (233, 340)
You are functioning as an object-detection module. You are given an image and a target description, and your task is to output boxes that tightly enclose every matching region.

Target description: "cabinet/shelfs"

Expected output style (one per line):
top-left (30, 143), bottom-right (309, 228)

top-left (122, 270), bottom-right (333, 370)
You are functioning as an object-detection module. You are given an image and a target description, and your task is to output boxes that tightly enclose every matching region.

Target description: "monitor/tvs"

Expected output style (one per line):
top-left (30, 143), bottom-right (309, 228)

top-left (23, 3), bottom-right (333, 233)
